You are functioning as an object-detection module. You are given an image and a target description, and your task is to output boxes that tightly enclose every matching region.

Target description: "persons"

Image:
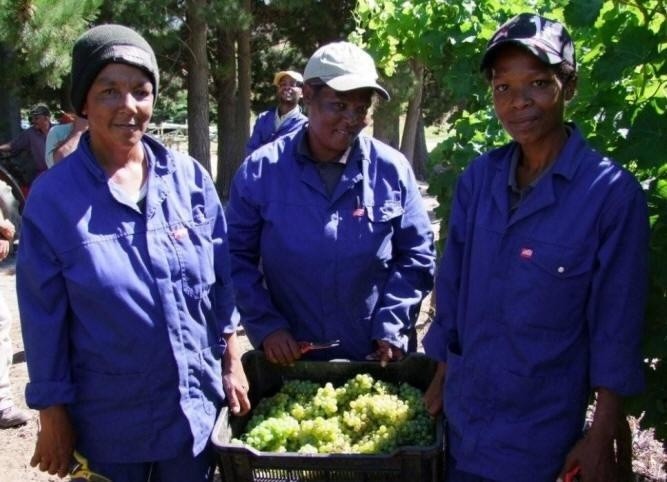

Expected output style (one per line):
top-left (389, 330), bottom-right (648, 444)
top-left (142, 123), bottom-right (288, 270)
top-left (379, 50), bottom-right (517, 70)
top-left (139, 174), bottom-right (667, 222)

top-left (44, 113), bottom-right (89, 170)
top-left (0, 103), bottom-right (53, 179)
top-left (222, 40), bottom-right (437, 369)
top-left (13, 24), bottom-right (252, 481)
top-left (242, 69), bottom-right (308, 159)
top-left (419, 11), bottom-right (650, 481)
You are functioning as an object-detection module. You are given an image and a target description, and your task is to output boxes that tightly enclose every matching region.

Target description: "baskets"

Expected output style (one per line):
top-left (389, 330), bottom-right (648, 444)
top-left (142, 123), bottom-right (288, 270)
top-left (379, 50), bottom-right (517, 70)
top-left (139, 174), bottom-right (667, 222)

top-left (210, 348), bottom-right (445, 482)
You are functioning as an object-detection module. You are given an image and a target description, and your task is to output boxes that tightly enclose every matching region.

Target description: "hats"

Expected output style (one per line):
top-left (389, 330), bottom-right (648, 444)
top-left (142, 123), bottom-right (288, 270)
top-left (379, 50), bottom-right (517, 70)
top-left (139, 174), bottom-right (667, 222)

top-left (71, 24), bottom-right (160, 119)
top-left (304, 41), bottom-right (391, 102)
top-left (30, 105), bottom-right (51, 116)
top-left (273, 70), bottom-right (304, 86)
top-left (480, 13), bottom-right (576, 74)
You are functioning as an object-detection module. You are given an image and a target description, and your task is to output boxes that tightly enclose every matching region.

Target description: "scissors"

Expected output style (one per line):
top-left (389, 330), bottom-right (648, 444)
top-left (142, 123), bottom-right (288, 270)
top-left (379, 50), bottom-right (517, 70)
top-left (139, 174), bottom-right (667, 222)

top-left (295, 339), bottom-right (340, 354)
top-left (66, 450), bottom-right (111, 482)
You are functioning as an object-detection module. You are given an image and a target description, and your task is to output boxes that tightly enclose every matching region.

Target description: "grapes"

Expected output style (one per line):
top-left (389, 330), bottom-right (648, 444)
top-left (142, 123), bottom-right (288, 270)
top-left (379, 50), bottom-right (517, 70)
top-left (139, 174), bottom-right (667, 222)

top-left (231, 372), bottom-right (435, 456)
